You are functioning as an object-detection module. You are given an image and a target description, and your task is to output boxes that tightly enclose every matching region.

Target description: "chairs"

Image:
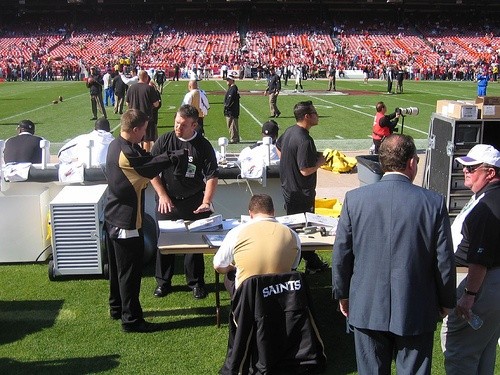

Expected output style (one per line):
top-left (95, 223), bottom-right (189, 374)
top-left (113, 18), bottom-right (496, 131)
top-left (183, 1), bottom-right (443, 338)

top-left (217, 271), bottom-right (328, 375)
top-left (0, 4), bottom-right (500, 79)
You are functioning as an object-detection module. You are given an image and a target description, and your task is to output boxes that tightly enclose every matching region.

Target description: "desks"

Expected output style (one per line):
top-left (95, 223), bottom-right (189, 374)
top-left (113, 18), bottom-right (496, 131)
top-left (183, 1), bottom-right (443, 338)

top-left (157, 218), bottom-right (336, 329)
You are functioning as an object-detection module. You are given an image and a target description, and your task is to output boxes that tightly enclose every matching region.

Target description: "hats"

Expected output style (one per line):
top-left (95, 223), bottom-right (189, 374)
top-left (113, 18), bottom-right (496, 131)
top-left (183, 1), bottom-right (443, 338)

top-left (454, 144), bottom-right (500, 168)
top-left (16, 119), bottom-right (35, 132)
top-left (262, 119), bottom-right (279, 137)
top-left (224, 75), bottom-right (235, 81)
top-left (90, 66), bottom-right (96, 70)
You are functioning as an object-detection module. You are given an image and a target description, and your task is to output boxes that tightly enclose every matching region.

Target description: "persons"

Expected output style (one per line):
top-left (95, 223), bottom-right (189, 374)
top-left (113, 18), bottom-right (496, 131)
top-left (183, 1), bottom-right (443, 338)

top-left (213, 194), bottom-right (302, 297)
top-left (332, 133), bottom-right (456, 374)
top-left (232, 31), bottom-right (326, 80)
top-left (373, 102), bottom-right (400, 154)
top-left (223, 75), bottom-right (241, 144)
top-left (4, 120), bottom-right (42, 163)
top-left (277, 101), bottom-right (329, 273)
top-left (395, 67), bottom-right (404, 94)
top-left (329, 27), bottom-right (419, 80)
top-left (126, 71), bottom-right (159, 152)
top-left (86, 64), bottom-right (166, 120)
top-left (477, 69), bottom-right (490, 95)
top-left (293, 62), bottom-right (304, 92)
top-left (58, 118), bottom-right (115, 167)
top-left (420, 40), bottom-right (500, 82)
top-left (121, 27), bottom-right (228, 79)
top-left (327, 64), bottom-right (336, 91)
top-left (103, 109), bottom-right (188, 333)
top-left (264, 67), bottom-right (281, 118)
top-left (183, 80), bottom-right (210, 133)
top-left (387, 67), bottom-right (393, 94)
top-left (238, 120), bottom-right (279, 161)
top-left (150, 104), bottom-right (217, 298)
top-left (5, 29), bottom-right (118, 81)
top-left (442, 144), bottom-right (500, 375)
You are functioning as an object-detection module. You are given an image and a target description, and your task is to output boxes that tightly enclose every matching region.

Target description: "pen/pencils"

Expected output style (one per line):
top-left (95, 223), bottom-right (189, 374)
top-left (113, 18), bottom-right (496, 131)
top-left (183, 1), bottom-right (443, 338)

top-left (299, 235), bottom-right (315, 238)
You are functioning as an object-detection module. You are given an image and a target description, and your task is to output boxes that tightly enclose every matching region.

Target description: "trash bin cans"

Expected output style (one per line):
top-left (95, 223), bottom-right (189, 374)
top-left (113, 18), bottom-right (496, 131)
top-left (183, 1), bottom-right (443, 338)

top-left (356, 155), bottom-right (384, 188)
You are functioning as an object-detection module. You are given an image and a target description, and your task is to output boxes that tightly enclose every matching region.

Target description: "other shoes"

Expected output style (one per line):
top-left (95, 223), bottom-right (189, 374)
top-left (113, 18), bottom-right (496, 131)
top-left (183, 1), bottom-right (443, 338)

top-left (228, 141), bottom-right (239, 144)
top-left (123, 321), bottom-right (159, 333)
top-left (193, 284), bottom-right (207, 297)
top-left (110, 311), bottom-right (122, 320)
top-left (153, 281), bottom-right (171, 296)
top-left (90, 117), bottom-right (97, 120)
top-left (275, 111), bottom-right (280, 118)
top-left (268, 115), bottom-right (275, 118)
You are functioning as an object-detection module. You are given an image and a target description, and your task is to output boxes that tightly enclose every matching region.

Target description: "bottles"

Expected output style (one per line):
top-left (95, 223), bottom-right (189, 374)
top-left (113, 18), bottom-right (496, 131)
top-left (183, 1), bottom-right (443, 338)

top-left (462, 309), bottom-right (483, 330)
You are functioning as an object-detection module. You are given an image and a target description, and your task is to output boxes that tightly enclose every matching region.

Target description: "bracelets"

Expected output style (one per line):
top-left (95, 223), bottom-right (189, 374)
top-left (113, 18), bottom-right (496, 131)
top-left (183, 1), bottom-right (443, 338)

top-left (464, 288), bottom-right (477, 296)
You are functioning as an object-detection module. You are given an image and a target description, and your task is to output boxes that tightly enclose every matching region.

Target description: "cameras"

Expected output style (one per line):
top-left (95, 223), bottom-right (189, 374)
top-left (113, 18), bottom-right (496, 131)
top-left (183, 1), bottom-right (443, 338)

top-left (395, 106), bottom-right (420, 115)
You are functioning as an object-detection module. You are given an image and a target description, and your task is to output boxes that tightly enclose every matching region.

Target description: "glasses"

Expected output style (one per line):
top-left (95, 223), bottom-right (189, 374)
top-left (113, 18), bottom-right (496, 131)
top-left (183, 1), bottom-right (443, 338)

top-left (462, 164), bottom-right (491, 173)
top-left (414, 157), bottom-right (420, 164)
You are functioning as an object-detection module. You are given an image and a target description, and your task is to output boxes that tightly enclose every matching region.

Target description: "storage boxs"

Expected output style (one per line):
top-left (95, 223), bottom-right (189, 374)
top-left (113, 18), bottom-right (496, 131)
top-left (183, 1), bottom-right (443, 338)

top-left (435, 96), bottom-right (500, 121)
